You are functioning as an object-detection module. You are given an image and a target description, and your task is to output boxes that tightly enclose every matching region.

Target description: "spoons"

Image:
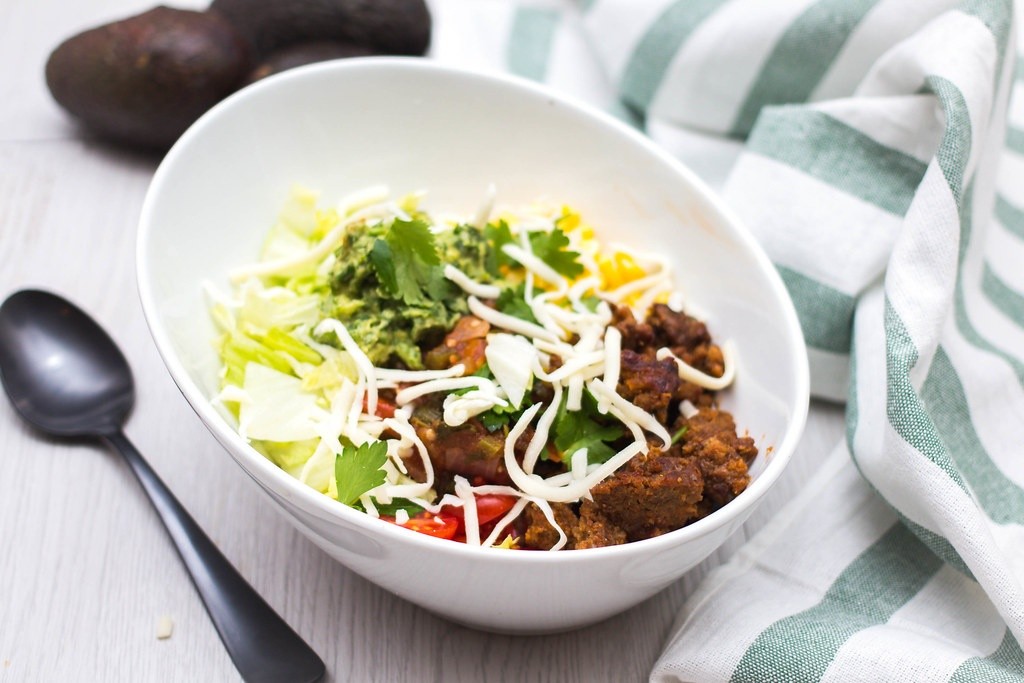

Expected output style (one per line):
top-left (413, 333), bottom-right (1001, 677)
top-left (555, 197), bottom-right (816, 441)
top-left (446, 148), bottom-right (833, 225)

top-left (0, 288), bottom-right (326, 683)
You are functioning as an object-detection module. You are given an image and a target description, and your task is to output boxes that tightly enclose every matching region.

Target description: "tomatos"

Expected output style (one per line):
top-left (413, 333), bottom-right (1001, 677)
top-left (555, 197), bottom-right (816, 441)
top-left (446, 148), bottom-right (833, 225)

top-left (379, 485), bottom-right (525, 550)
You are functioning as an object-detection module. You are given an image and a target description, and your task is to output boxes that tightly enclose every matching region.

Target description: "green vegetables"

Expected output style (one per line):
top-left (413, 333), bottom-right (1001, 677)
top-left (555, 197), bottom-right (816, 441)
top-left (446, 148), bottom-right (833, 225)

top-left (217, 190), bottom-right (687, 512)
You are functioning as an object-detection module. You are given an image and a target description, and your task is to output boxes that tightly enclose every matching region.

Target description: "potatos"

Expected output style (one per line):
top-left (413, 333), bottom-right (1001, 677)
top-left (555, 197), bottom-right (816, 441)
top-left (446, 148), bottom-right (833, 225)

top-left (45, 0), bottom-right (433, 155)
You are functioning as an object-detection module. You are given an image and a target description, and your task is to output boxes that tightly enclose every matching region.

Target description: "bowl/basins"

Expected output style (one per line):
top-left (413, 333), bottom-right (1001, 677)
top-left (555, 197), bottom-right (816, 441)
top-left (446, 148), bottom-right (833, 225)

top-left (136, 55), bottom-right (809, 634)
